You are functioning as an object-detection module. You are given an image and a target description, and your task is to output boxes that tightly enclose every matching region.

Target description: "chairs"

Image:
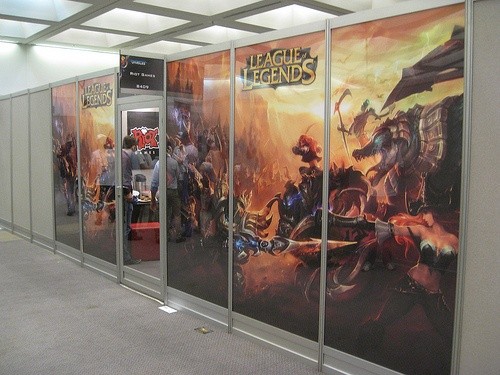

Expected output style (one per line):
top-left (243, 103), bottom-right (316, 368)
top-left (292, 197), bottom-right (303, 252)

top-left (132, 174), bottom-right (146, 199)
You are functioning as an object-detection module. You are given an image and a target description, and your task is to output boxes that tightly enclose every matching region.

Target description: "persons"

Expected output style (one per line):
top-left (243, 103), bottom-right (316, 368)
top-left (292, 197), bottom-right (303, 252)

top-left (122, 135), bottom-right (146, 242)
top-left (174, 133), bottom-right (224, 239)
top-left (56, 134), bottom-right (79, 217)
top-left (150, 145), bottom-right (186, 244)
top-left (92, 138), bottom-right (115, 225)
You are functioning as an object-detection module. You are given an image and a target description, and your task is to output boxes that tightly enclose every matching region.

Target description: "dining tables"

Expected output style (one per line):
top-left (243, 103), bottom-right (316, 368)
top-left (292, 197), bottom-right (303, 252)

top-left (132, 198), bottom-right (159, 223)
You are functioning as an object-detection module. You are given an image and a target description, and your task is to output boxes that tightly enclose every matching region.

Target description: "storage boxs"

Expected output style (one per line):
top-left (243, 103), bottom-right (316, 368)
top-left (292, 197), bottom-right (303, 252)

top-left (128, 221), bottom-right (161, 261)
